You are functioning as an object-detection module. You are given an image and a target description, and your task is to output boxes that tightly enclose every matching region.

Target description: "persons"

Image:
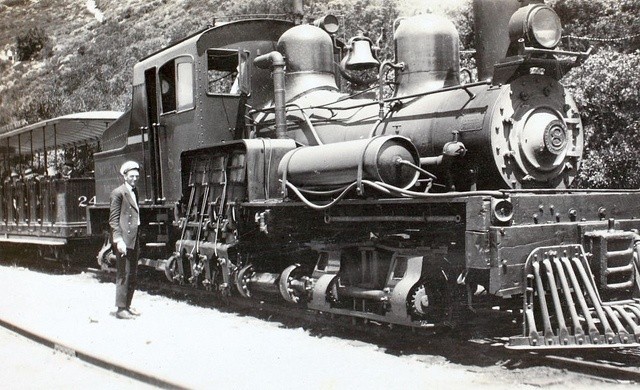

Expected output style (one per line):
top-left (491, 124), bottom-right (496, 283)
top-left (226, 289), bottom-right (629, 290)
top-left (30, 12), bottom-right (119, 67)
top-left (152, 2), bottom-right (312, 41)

top-left (1, 160), bottom-right (81, 221)
top-left (107, 159), bottom-right (142, 321)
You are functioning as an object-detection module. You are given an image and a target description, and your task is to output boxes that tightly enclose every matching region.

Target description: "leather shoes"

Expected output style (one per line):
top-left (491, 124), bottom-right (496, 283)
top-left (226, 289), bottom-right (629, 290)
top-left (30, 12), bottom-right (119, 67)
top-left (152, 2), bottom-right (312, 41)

top-left (128, 308), bottom-right (141, 316)
top-left (116, 309), bottom-right (140, 320)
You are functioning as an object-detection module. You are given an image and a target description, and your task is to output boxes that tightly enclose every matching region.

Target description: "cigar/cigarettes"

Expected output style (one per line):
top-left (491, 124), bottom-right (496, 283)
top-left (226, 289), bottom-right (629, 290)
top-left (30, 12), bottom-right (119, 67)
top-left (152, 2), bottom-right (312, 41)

top-left (121, 250), bottom-right (127, 258)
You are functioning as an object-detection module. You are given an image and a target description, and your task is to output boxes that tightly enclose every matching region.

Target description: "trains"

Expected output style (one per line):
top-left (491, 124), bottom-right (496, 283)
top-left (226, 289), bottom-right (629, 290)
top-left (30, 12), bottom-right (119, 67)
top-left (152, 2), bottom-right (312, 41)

top-left (0, 0), bottom-right (640, 350)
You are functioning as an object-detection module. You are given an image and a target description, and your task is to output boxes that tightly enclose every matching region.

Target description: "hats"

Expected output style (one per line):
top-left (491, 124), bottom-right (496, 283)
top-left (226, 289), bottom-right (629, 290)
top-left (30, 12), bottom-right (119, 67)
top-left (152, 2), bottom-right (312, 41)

top-left (120, 160), bottom-right (140, 175)
